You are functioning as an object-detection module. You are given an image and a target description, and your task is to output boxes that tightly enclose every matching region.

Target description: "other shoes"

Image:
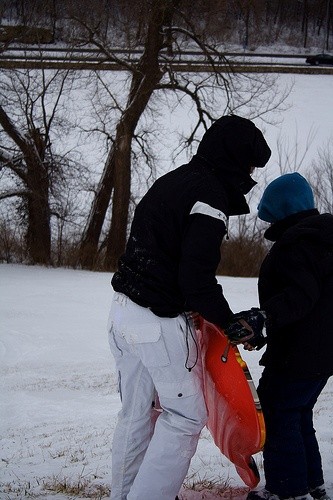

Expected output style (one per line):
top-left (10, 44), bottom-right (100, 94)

top-left (246, 482), bottom-right (327, 500)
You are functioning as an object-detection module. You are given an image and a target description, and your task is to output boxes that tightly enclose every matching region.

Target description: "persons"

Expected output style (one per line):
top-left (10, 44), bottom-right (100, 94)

top-left (239, 172), bottom-right (333, 500)
top-left (97, 115), bottom-right (272, 500)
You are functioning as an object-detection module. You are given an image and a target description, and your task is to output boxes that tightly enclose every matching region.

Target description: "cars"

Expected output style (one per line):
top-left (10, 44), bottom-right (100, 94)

top-left (304, 54), bottom-right (333, 66)
